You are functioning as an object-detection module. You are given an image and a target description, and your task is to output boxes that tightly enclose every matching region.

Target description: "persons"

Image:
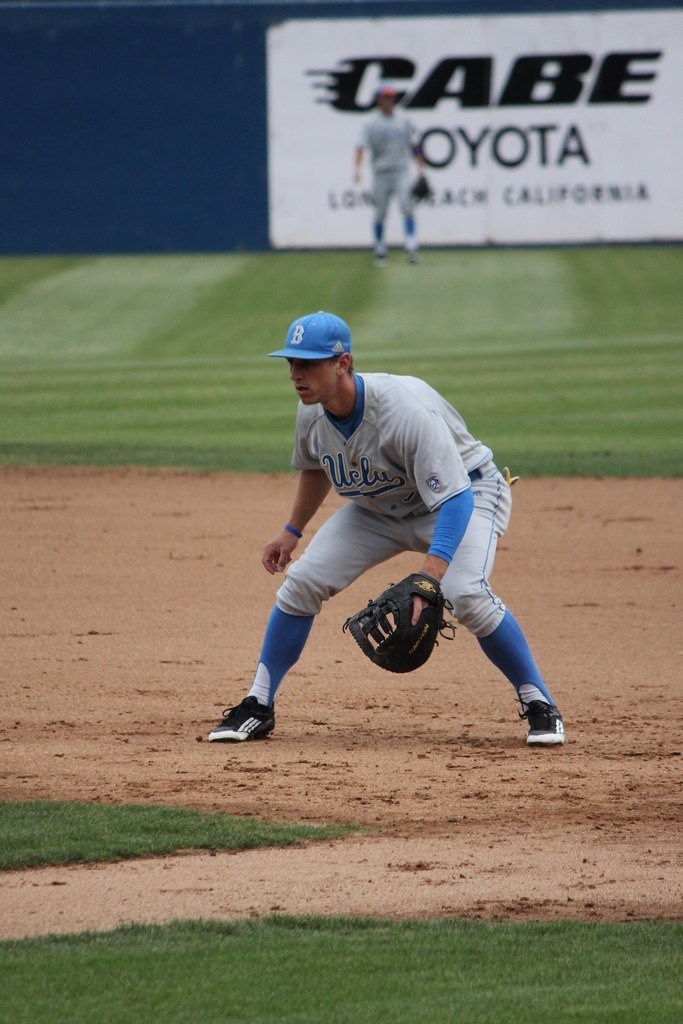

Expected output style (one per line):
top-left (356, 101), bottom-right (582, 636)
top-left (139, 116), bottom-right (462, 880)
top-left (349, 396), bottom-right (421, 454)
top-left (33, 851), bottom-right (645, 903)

top-left (351, 84), bottom-right (428, 268)
top-left (207, 308), bottom-right (569, 746)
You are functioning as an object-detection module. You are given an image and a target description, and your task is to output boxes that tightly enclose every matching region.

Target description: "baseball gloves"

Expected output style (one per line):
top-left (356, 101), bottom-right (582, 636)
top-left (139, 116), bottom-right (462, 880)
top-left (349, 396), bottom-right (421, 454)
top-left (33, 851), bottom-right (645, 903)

top-left (340, 571), bottom-right (458, 674)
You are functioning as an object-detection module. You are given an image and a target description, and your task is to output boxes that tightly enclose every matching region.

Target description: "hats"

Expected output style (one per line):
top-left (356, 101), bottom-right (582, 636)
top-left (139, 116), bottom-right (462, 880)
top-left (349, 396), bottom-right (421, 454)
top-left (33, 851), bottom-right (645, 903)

top-left (374, 86), bottom-right (393, 97)
top-left (268, 310), bottom-right (352, 359)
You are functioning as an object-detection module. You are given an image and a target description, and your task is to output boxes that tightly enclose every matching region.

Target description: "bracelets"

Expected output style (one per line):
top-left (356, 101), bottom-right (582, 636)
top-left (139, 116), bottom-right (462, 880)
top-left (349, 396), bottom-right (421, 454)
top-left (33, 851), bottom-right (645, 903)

top-left (284, 524), bottom-right (302, 538)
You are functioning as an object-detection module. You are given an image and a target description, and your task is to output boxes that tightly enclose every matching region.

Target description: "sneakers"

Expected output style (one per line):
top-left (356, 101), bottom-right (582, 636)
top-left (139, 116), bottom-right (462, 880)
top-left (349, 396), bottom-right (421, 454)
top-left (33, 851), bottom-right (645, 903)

top-left (208, 696), bottom-right (276, 742)
top-left (525, 702), bottom-right (566, 745)
